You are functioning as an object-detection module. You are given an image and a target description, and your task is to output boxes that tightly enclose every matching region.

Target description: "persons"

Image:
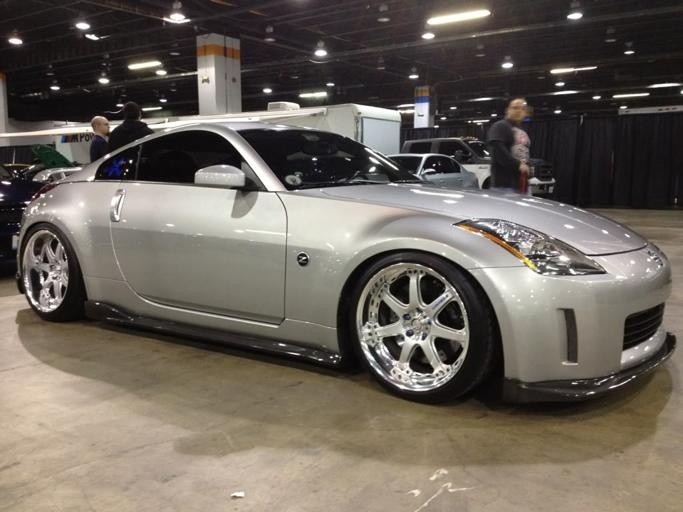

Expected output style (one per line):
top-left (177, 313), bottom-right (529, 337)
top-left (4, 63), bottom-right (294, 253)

top-left (482, 97), bottom-right (533, 195)
top-left (108, 100), bottom-right (152, 149)
top-left (87, 115), bottom-right (111, 164)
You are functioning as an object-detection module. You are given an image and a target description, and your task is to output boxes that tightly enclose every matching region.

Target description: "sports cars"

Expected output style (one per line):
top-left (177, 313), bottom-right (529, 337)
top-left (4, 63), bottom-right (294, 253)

top-left (17, 122), bottom-right (678, 413)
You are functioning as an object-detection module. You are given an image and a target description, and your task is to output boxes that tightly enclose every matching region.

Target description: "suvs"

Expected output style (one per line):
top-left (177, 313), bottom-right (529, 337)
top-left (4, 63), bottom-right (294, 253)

top-left (400, 136), bottom-right (557, 197)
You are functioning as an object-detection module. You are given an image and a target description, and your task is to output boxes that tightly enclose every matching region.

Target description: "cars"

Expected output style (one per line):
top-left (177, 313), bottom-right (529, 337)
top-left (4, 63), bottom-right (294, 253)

top-left (0, 162), bottom-right (82, 276)
top-left (367, 152), bottom-right (478, 193)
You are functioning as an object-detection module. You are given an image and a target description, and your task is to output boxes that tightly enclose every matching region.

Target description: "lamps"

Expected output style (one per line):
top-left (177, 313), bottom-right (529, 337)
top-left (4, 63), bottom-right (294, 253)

top-left (7, 1), bottom-right (186, 85)
